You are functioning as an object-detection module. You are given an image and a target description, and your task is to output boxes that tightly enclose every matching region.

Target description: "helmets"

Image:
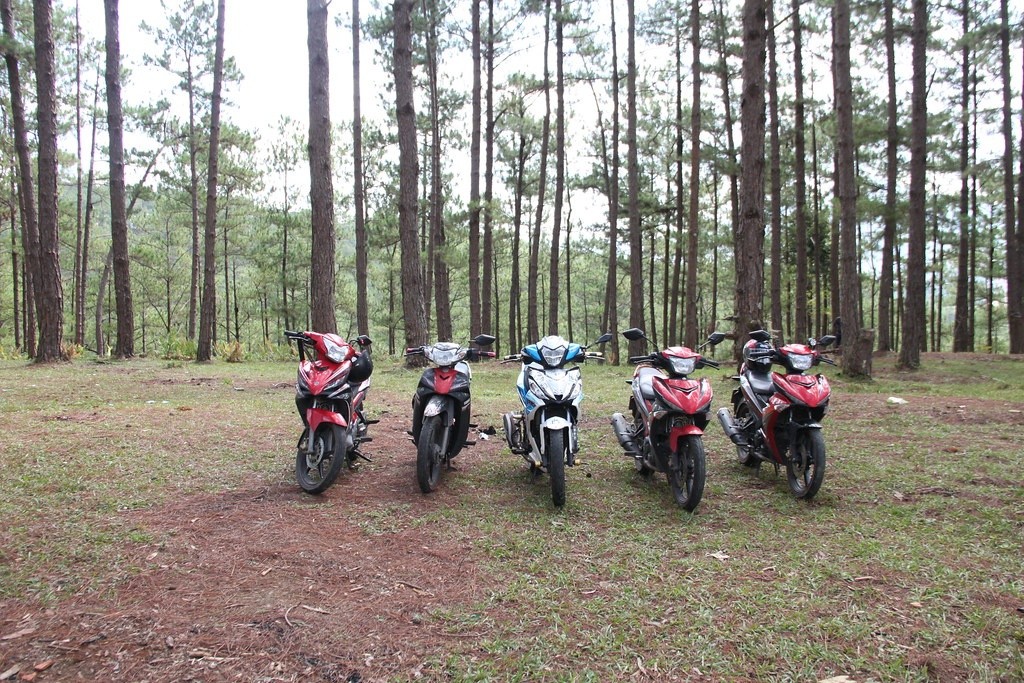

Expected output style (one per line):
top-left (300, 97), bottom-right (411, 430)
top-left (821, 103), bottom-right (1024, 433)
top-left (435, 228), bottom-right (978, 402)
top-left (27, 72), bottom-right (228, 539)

top-left (347, 349), bottom-right (373, 383)
top-left (743, 338), bottom-right (774, 373)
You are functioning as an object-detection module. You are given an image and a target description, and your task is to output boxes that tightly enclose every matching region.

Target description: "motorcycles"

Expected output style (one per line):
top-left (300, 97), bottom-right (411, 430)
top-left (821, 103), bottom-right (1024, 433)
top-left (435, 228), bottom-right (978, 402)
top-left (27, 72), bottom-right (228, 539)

top-left (500, 332), bottom-right (612, 507)
top-left (718, 330), bottom-right (840, 500)
top-left (404, 334), bottom-right (497, 492)
top-left (610, 328), bottom-right (725, 513)
top-left (283, 329), bottom-right (380, 496)
